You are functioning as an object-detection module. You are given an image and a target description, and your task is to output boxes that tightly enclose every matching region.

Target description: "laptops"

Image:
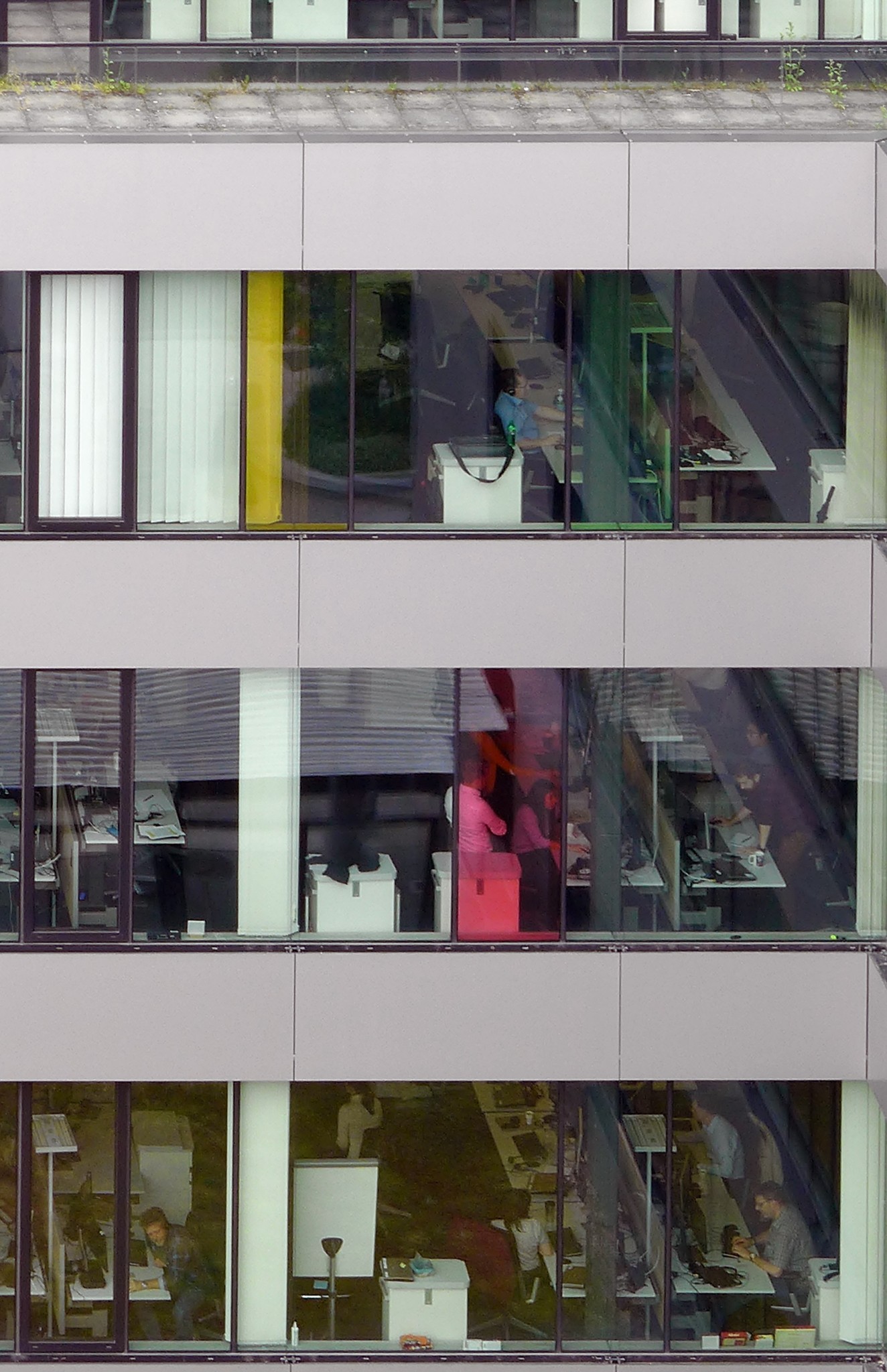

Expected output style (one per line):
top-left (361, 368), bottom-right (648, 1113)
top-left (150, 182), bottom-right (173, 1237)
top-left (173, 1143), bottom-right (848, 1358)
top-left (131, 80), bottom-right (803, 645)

top-left (562, 1265), bottom-right (587, 1288)
top-left (549, 1229), bottom-right (583, 1257)
top-left (529, 1173), bottom-right (557, 1193)
top-left (698, 1267), bottom-right (740, 1289)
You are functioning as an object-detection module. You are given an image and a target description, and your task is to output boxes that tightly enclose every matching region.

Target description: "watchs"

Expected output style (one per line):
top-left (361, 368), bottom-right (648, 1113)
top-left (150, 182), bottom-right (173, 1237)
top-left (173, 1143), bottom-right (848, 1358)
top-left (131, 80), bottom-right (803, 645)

top-left (749, 1253), bottom-right (758, 1262)
top-left (141, 1280), bottom-right (148, 1289)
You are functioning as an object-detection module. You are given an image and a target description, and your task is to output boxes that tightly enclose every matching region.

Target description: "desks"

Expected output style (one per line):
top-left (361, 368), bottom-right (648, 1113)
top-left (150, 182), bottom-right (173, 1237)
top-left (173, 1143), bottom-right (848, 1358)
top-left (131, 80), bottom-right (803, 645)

top-left (512, 672), bottom-right (790, 887)
top-left (70, 770), bottom-right (188, 846)
top-left (450, 270), bottom-right (777, 484)
top-left (0, 797), bottom-right (56, 883)
top-left (473, 1081), bottom-right (778, 1297)
top-left (0, 1103), bottom-right (172, 1298)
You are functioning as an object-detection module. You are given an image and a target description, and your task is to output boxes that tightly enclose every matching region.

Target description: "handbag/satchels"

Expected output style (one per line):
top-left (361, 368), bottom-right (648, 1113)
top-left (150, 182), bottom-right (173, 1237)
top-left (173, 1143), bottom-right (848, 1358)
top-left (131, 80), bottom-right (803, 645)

top-left (701, 1266), bottom-right (742, 1290)
top-left (449, 433), bottom-right (514, 483)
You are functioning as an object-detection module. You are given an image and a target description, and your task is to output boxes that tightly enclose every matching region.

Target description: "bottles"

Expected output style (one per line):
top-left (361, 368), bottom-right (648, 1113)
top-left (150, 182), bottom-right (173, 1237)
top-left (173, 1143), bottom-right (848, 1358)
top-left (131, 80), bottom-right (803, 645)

top-left (555, 388), bottom-right (566, 411)
top-left (291, 1321), bottom-right (298, 1346)
top-left (507, 421), bottom-right (516, 446)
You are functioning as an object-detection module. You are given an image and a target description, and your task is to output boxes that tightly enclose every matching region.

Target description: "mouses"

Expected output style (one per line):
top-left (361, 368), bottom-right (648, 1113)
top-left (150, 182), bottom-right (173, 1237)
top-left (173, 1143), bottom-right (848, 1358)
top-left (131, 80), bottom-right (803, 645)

top-left (724, 1225), bottom-right (737, 1229)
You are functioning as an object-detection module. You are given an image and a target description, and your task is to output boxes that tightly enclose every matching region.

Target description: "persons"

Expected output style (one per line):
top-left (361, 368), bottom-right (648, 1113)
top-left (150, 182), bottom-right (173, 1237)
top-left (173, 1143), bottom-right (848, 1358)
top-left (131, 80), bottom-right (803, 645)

top-left (335, 1081), bottom-right (383, 1158)
top-left (127, 1206), bottom-right (207, 1340)
top-left (495, 369), bottom-right (583, 519)
top-left (444, 732), bottom-right (590, 890)
top-left (672, 1095), bottom-right (745, 1200)
top-left (729, 1180), bottom-right (815, 1307)
top-left (490, 1188), bottom-right (553, 1291)
top-left (708, 720), bottom-right (806, 870)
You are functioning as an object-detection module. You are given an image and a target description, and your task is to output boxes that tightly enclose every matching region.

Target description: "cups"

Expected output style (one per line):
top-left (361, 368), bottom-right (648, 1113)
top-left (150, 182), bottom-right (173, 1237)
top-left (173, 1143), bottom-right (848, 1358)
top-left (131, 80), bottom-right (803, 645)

top-left (748, 851), bottom-right (764, 867)
top-left (525, 1111), bottom-right (533, 1124)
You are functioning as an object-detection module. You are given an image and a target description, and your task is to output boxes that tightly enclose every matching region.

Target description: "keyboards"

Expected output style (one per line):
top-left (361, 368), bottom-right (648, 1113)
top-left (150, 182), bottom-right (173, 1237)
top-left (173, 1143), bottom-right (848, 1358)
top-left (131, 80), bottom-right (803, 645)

top-left (722, 1229), bottom-right (741, 1261)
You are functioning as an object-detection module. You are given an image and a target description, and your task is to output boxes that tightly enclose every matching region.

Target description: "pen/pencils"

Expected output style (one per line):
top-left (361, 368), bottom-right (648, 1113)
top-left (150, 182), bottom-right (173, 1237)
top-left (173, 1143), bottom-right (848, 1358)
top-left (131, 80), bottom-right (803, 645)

top-left (144, 795), bottom-right (154, 802)
top-left (742, 836), bottom-right (752, 843)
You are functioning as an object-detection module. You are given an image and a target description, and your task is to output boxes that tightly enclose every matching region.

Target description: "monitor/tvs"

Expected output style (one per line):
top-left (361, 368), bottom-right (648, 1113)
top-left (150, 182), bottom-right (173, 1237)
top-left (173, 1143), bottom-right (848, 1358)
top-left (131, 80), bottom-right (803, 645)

top-left (685, 1190), bottom-right (707, 1255)
top-left (681, 1153), bottom-right (692, 1209)
top-left (77, 1213), bottom-right (108, 1275)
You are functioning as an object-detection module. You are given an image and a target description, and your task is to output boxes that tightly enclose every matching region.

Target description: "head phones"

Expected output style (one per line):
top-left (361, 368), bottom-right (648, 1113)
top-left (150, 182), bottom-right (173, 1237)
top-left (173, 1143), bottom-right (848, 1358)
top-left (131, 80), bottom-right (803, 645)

top-left (508, 1156), bottom-right (527, 1169)
